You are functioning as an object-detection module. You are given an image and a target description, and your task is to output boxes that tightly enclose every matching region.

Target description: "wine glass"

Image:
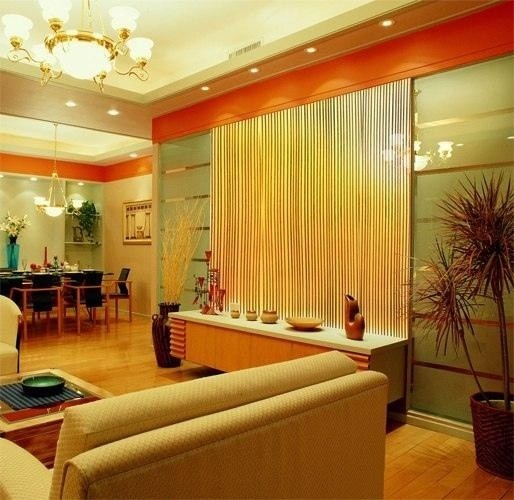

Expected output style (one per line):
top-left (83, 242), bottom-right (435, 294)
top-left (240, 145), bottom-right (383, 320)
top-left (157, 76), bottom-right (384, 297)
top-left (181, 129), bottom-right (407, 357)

top-left (22, 259), bottom-right (27, 273)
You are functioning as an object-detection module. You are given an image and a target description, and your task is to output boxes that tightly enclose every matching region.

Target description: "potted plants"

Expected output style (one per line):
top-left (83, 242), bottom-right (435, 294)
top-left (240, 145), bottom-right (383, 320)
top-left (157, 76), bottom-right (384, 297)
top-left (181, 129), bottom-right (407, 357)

top-left (395, 170), bottom-right (514, 481)
top-left (67, 199), bottom-right (96, 242)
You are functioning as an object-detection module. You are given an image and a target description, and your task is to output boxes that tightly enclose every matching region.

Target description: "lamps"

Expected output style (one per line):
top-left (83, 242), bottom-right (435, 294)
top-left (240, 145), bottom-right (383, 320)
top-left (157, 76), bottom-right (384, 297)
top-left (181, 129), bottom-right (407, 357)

top-left (34, 122), bottom-right (82, 218)
top-left (192, 250), bottom-right (225, 315)
top-left (1, 0), bottom-right (154, 96)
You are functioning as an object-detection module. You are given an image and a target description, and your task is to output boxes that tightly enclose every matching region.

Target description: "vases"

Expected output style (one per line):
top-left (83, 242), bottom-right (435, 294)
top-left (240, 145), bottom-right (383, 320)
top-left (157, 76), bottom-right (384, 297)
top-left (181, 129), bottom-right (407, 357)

top-left (152, 303), bottom-right (182, 368)
top-left (6, 236), bottom-right (20, 270)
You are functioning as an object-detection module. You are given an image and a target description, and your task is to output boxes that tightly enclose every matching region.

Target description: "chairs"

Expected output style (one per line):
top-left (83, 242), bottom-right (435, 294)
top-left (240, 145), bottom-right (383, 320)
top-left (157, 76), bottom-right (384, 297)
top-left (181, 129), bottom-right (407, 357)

top-left (0, 268), bottom-right (132, 342)
top-left (0, 295), bottom-right (25, 376)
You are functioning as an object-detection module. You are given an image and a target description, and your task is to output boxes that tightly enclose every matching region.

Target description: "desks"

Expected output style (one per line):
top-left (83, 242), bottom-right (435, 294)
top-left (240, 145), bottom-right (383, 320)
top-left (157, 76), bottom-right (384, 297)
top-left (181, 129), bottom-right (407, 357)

top-left (168, 310), bottom-right (407, 403)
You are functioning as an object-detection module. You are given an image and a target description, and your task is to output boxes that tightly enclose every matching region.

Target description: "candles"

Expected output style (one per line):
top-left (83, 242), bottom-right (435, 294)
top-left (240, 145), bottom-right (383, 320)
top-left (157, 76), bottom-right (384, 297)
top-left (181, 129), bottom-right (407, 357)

top-left (44, 247), bottom-right (47, 268)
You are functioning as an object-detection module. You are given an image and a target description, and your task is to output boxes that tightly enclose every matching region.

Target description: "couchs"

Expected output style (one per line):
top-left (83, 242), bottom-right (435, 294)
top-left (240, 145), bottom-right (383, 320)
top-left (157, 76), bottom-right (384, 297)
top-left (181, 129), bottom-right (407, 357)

top-left (0, 350), bottom-right (389, 500)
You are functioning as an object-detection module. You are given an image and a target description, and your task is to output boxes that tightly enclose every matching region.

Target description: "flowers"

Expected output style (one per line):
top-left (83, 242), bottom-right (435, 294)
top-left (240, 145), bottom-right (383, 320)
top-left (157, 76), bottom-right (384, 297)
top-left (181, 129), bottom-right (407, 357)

top-left (0, 208), bottom-right (31, 267)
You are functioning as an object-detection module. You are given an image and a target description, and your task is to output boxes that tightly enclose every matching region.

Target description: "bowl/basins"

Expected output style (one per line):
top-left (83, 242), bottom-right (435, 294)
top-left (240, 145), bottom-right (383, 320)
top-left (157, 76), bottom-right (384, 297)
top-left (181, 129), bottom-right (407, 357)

top-left (285, 317), bottom-right (324, 331)
top-left (22, 375), bottom-right (66, 396)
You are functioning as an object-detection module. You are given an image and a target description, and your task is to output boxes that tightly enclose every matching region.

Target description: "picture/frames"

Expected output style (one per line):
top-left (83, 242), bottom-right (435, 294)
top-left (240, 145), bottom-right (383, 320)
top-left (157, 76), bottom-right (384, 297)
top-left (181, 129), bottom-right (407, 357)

top-left (122, 199), bottom-right (152, 245)
